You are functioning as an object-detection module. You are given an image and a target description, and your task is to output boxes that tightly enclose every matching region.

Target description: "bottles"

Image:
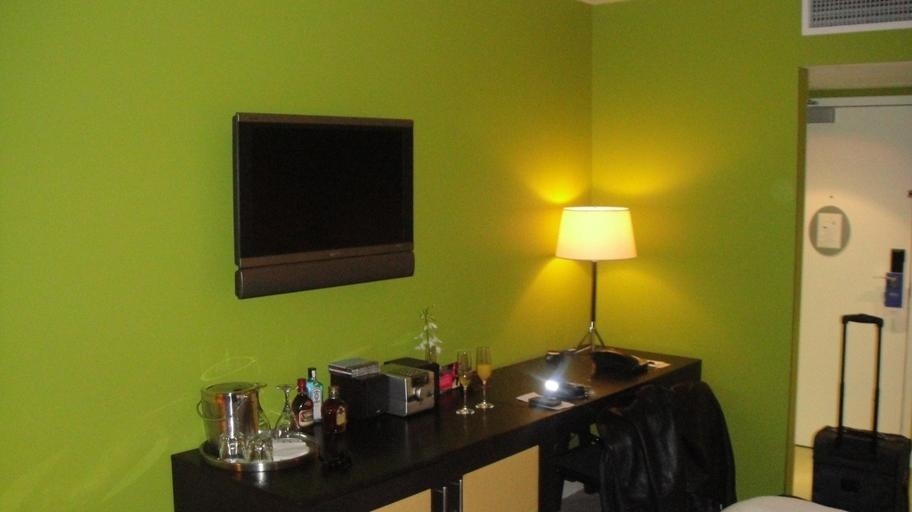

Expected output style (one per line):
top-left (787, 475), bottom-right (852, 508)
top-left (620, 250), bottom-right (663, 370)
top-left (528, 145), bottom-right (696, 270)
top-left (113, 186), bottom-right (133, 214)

top-left (291, 367), bottom-right (348, 435)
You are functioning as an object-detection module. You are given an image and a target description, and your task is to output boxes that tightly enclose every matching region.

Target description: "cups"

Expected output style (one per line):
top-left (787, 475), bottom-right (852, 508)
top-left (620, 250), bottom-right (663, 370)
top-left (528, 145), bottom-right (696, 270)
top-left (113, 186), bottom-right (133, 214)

top-left (218, 432), bottom-right (274, 462)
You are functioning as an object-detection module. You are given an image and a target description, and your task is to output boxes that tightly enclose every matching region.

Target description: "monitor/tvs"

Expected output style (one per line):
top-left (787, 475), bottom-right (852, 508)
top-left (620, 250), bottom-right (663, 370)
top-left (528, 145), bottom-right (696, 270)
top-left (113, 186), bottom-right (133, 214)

top-left (232, 112), bottom-right (414, 270)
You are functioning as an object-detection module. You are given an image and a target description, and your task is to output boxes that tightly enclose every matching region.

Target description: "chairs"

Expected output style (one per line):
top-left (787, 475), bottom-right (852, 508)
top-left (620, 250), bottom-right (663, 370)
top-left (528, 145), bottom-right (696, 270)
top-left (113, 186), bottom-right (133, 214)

top-left (547, 379), bottom-right (708, 512)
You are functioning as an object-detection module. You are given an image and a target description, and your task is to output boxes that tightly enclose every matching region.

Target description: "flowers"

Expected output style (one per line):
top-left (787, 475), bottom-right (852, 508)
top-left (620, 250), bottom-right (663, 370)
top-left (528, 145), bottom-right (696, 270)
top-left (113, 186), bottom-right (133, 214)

top-left (412, 302), bottom-right (443, 362)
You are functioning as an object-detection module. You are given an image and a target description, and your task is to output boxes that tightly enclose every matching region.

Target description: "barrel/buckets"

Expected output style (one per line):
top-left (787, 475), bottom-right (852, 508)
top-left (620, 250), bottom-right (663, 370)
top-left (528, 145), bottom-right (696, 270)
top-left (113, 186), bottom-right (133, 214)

top-left (198, 382), bottom-right (267, 447)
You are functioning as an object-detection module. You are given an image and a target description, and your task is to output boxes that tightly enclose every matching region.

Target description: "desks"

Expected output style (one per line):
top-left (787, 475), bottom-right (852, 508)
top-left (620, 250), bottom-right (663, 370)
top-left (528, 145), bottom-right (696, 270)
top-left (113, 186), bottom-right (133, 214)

top-left (171, 342), bottom-right (702, 511)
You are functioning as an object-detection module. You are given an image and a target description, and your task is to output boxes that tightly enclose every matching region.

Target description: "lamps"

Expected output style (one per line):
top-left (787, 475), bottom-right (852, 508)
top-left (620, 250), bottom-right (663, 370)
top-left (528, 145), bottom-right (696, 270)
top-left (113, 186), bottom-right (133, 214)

top-left (555, 206), bottom-right (638, 354)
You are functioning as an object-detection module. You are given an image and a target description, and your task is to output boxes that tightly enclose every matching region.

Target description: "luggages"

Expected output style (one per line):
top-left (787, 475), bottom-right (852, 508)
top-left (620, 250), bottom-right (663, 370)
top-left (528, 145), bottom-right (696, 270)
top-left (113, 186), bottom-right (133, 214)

top-left (811, 314), bottom-right (912, 512)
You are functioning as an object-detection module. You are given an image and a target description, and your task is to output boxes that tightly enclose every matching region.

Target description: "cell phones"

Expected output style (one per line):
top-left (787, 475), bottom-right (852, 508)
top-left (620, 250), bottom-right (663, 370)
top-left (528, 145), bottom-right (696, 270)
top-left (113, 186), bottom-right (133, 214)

top-left (531, 397), bottom-right (561, 407)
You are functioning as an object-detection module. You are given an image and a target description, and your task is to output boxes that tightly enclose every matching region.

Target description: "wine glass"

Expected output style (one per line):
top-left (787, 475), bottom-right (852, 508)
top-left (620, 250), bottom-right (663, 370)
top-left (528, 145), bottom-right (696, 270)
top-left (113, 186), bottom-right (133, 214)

top-left (252, 381), bottom-right (301, 443)
top-left (456, 351), bottom-right (476, 416)
top-left (475, 346), bottom-right (496, 411)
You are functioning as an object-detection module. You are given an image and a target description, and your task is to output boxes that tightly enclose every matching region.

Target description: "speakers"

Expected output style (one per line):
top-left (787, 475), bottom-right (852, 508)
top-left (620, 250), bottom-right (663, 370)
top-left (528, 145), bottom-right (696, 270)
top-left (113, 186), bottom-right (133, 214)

top-left (331, 373), bottom-right (390, 420)
top-left (384, 358), bottom-right (440, 407)
top-left (235, 251), bottom-right (414, 299)
